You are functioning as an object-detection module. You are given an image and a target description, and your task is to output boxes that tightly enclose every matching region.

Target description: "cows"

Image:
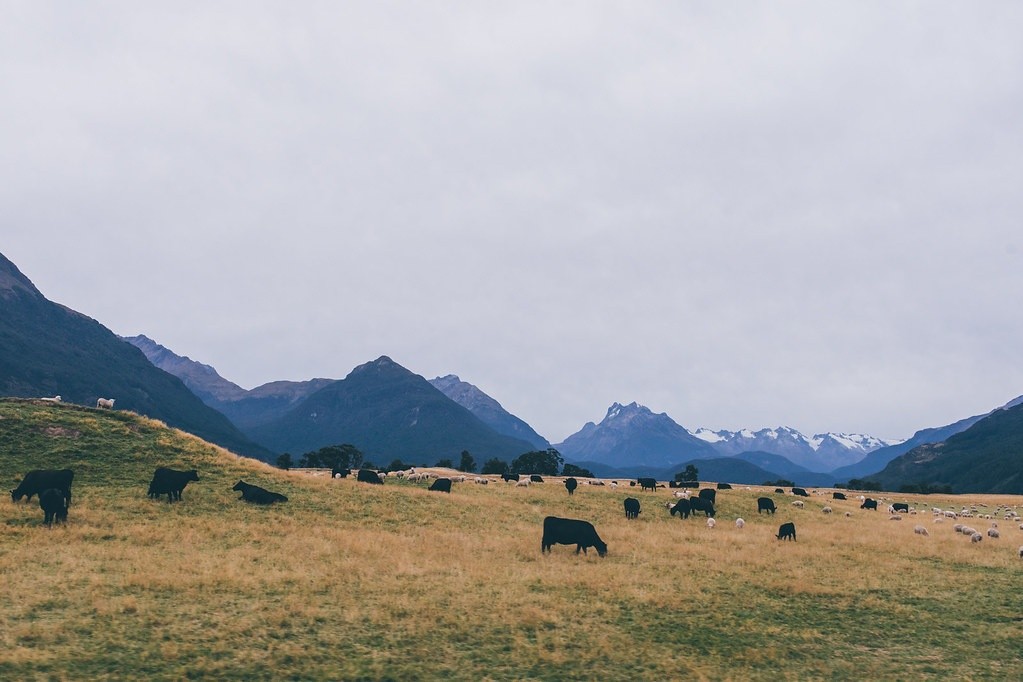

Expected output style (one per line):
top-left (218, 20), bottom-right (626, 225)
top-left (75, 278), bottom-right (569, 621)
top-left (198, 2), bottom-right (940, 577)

top-left (428, 477), bottom-right (451, 493)
top-left (758, 497), bottom-right (777, 514)
top-left (775, 488), bottom-right (784, 494)
top-left (500, 472), bottom-right (544, 483)
top-left (892, 502), bottom-right (909, 512)
top-left (792, 487), bottom-right (808, 496)
top-left (624, 497), bottom-right (642, 519)
top-left (669, 488), bottom-right (716, 518)
top-left (148, 467), bottom-right (199, 502)
top-left (775, 521), bottom-right (797, 541)
top-left (331, 467), bottom-right (352, 478)
top-left (10, 469), bottom-right (75, 526)
top-left (860, 498), bottom-right (877, 511)
top-left (356, 469), bottom-right (384, 484)
top-left (232, 481), bottom-right (287, 505)
top-left (637, 477), bottom-right (733, 490)
top-left (833, 492), bottom-right (846, 500)
top-left (541, 516), bottom-right (608, 558)
top-left (563, 477), bottom-right (577, 495)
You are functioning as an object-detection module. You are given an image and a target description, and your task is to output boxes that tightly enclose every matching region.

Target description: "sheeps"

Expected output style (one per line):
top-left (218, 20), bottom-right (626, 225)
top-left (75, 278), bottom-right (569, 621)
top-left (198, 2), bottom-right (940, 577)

top-left (335, 468), bottom-right (496, 484)
top-left (791, 489), bottom-right (1023, 558)
top-left (665, 491), bottom-right (744, 528)
top-left (580, 478), bottom-right (619, 487)
top-left (515, 479), bottom-right (531, 487)
